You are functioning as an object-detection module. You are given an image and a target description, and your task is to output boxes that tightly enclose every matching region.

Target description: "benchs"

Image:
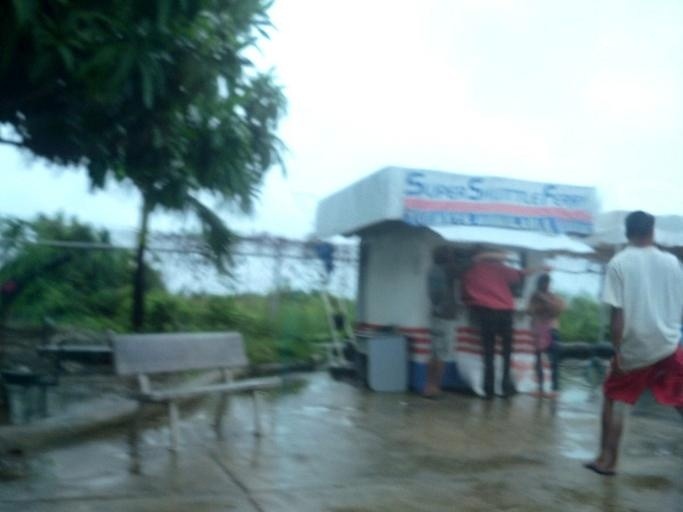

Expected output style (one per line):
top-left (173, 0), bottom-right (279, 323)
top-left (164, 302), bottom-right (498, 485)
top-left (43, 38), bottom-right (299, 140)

top-left (102, 326), bottom-right (288, 455)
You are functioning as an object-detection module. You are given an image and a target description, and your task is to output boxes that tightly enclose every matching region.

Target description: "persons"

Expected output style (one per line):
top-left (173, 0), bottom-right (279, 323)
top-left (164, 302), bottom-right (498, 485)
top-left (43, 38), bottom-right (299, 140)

top-left (425, 243), bottom-right (525, 398)
top-left (584, 210), bottom-right (683, 475)
top-left (527, 273), bottom-right (567, 395)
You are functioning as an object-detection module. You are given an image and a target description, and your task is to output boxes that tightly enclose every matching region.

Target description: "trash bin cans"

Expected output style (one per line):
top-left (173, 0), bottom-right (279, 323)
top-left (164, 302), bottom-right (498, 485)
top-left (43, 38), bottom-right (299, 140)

top-left (5, 372), bottom-right (48, 425)
top-left (368, 334), bottom-right (407, 394)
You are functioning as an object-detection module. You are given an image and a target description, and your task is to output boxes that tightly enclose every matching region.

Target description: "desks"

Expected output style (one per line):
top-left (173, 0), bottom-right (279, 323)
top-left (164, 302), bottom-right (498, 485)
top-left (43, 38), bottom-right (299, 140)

top-left (351, 331), bottom-right (412, 396)
